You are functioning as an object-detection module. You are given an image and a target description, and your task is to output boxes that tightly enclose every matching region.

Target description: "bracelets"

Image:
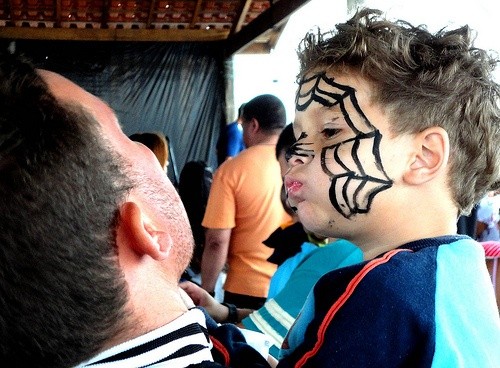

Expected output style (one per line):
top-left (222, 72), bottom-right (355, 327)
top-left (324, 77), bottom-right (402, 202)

top-left (209, 291), bottom-right (215, 297)
top-left (222, 302), bottom-right (238, 323)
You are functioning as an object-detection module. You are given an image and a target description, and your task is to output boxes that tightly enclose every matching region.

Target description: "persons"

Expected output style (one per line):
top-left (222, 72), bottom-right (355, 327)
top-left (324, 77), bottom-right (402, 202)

top-left (201, 95), bottom-right (292, 310)
top-left (0, 51), bottom-right (222, 368)
top-left (178, 122), bottom-right (362, 367)
top-left (217, 103), bottom-right (247, 167)
top-left (179, 9), bottom-right (500, 368)
